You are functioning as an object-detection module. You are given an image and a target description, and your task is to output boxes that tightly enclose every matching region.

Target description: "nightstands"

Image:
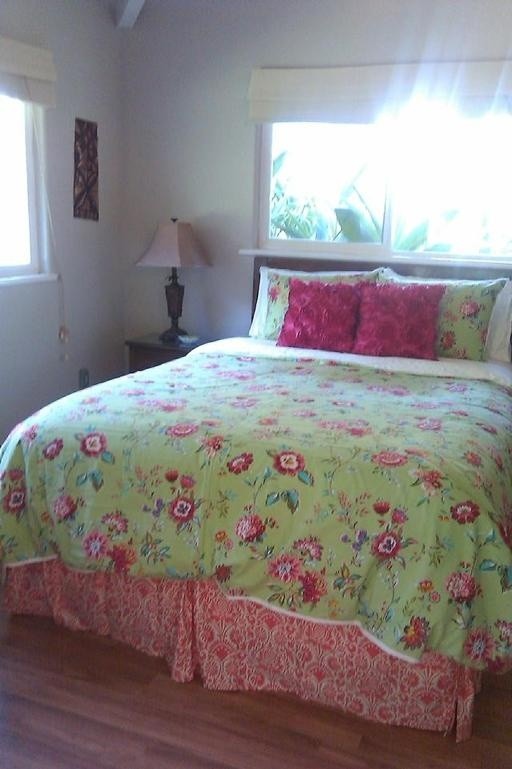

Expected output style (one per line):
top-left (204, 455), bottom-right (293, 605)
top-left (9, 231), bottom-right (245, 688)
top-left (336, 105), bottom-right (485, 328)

top-left (125, 331), bottom-right (219, 375)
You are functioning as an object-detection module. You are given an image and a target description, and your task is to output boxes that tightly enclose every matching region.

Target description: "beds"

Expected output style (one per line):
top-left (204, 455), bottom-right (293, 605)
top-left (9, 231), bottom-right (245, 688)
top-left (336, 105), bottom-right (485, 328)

top-left (0, 255), bottom-right (512, 745)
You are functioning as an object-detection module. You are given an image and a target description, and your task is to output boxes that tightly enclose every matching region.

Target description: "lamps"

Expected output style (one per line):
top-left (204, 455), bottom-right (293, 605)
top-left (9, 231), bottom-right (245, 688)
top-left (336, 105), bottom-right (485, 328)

top-left (135, 215), bottom-right (213, 342)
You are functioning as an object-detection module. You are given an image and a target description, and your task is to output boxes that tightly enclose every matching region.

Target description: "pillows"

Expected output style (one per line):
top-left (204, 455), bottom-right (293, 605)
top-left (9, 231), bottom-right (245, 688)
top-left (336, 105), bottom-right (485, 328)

top-left (276, 276), bottom-right (363, 352)
top-left (353, 281), bottom-right (446, 361)
top-left (256, 266), bottom-right (384, 339)
top-left (487, 281), bottom-right (512, 362)
top-left (377, 267), bottom-right (507, 362)
top-left (248, 266), bottom-right (366, 338)
